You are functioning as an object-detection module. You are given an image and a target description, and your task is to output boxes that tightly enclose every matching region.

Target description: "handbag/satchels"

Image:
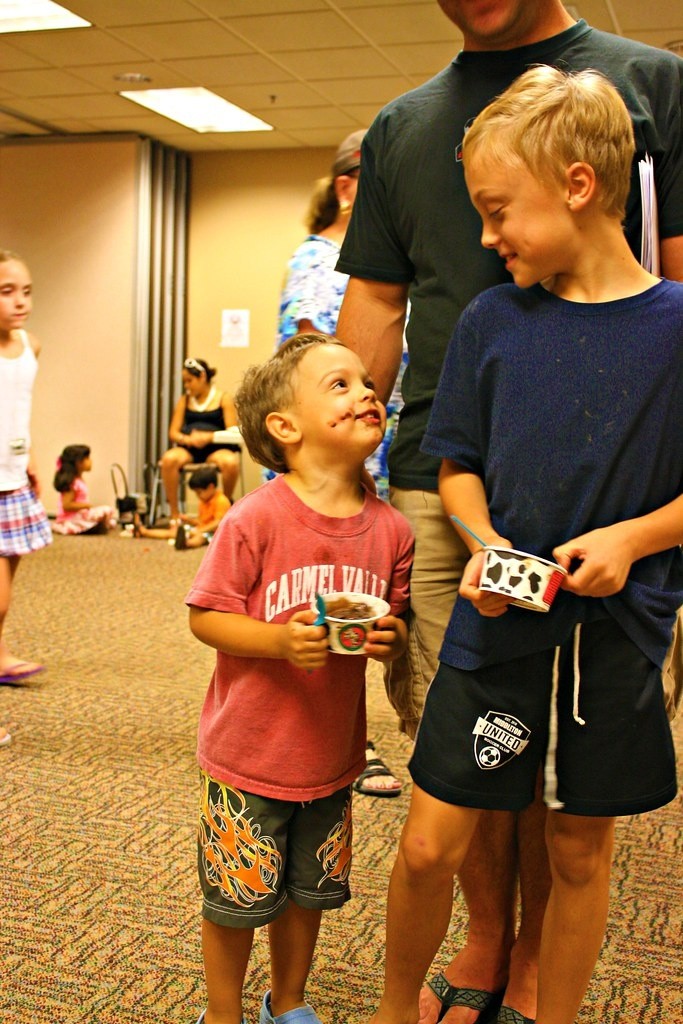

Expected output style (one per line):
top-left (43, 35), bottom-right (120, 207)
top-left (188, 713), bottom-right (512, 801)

top-left (111, 463), bottom-right (149, 525)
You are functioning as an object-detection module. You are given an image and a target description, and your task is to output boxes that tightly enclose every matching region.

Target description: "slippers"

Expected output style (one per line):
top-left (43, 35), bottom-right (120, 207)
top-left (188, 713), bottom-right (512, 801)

top-left (418, 970), bottom-right (505, 1024)
top-left (493, 1004), bottom-right (534, 1024)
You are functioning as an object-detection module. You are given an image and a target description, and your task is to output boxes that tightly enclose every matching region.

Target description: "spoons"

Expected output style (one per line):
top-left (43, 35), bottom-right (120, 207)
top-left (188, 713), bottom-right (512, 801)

top-left (307, 592), bottom-right (327, 674)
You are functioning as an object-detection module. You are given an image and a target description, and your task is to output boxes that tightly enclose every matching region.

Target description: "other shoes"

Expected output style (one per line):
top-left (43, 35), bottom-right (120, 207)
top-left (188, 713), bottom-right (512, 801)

top-left (0, 658), bottom-right (43, 682)
top-left (0, 727), bottom-right (11, 748)
top-left (133, 512), bottom-right (143, 537)
top-left (175, 526), bottom-right (187, 549)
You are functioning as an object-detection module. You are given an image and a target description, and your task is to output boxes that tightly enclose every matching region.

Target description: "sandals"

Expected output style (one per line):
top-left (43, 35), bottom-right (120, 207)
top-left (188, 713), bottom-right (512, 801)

top-left (260, 989), bottom-right (323, 1024)
top-left (196, 1008), bottom-right (247, 1024)
top-left (351, 740), bottom-right (402, 795)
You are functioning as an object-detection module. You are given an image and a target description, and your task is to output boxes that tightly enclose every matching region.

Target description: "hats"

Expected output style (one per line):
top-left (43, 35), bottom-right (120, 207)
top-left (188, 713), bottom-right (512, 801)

top-left (332, 129), bottom-right (371, 174)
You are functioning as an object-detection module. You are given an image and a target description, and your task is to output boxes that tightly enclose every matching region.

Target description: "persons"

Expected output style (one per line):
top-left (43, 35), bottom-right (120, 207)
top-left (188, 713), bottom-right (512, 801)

top-left (182, 331), bottom-right (417, 1024)
top-left (1, 126), bottom-right (429, 800)
top-left (364, 66), bottom-right (683, 1024)
top-left (328, 1), bottom-right (683, 1024)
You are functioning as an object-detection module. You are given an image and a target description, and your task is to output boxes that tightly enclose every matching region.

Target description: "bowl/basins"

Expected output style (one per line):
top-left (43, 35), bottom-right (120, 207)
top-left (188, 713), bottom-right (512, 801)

top-left (311, 591), bottom-right (391, 656)
top-left (478, 546), bottom-right (568, 613)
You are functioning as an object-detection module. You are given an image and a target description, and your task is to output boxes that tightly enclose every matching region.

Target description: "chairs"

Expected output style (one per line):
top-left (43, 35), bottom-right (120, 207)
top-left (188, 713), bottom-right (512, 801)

top-left (148, 447), bottom-right (245, 530)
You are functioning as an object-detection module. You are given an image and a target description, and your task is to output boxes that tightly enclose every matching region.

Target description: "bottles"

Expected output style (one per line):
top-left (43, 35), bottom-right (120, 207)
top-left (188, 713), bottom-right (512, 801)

top-left (166, 513), bottom-right (183, 545)
